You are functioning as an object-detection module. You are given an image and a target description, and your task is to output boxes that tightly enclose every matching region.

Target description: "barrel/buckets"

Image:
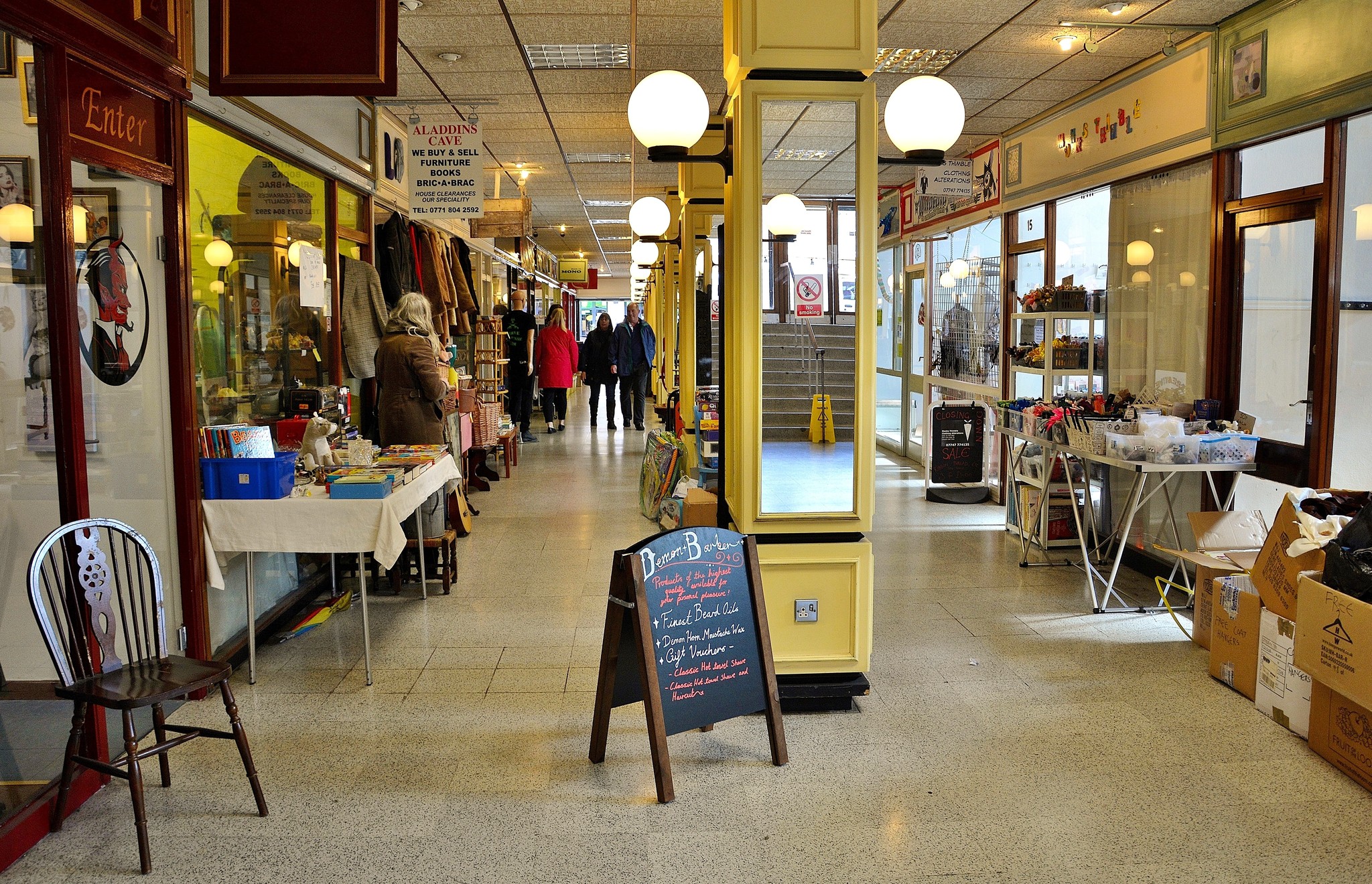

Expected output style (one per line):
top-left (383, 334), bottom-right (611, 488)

top-left (402, 486), bottom-right (446, 539)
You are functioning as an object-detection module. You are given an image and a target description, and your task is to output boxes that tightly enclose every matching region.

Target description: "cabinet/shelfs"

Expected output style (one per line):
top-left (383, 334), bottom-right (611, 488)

top-left (477, 318), bottom-right (511, 411)
top-left (1006, 312), bottom-right (1094, 549)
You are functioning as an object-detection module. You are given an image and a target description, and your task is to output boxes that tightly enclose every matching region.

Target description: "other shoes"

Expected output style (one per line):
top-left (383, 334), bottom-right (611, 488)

top-left (547, 427), bottom-right (556, 433)
top-left (558, 424), bottom-right (565, 431)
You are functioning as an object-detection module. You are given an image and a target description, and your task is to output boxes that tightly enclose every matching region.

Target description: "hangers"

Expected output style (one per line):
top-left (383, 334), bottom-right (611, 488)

top-left (383, 201), bottom-right (471, 249)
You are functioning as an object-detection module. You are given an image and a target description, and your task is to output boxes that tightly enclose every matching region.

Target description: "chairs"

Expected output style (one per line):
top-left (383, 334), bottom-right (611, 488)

top-left (24, 515), bottom-right (270, 876)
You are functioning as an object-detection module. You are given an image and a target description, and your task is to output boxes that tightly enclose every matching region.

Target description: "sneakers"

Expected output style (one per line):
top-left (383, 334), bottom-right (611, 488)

top-left (521, 427), bottom-right (538, 442)
top-left (623, 418), bottom-right (631, 427)
top-left (590, 417), bottom-right (597, 426)
top-left (607, 421), bottom-right (617, 430)
top-left (635, 422), bottom-right (645, 431)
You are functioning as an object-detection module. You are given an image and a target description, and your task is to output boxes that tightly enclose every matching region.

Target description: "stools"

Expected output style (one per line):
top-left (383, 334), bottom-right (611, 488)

top-left (351, 530), bottom-right (457, 595)
top-left (494, 426), bottom-right (519, 476)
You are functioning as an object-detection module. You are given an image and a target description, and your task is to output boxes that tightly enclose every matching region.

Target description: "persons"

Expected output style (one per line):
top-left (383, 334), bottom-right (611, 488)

top-left (273, 295), bottom-right (320, 341)
top-left (534, 308), bottom-right (579, 434)
top-left (502, 290), bottom-right (538, 442)
top-left (580, 312), bottom-right (618, 430)
top-left (373, 291), bottom-right (450, 576)
top-left (608, 303), bottom-right (656, 430)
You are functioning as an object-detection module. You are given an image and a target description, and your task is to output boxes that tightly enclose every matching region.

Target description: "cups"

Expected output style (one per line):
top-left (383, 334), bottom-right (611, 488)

top-left (347, 435), bottom-right (372, 466)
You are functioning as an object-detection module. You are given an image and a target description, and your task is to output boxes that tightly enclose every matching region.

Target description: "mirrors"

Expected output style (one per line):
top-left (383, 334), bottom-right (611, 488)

top-left (744, 83), bottom-right (876, 542)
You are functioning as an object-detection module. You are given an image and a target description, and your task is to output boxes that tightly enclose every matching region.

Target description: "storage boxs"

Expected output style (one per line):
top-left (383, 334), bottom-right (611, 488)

top-left (996, 405), bottom-right (1260, 463)
top-left (1011, 451), bottom-right (1084, 483)
top-left (1154, 505), bottom-right (1372, 791)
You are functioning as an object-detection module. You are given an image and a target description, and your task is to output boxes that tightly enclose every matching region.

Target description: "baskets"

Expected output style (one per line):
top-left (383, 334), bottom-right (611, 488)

top-left (1041, 291), bottom-right (1087, 311)
top-left (442, 386), bottom-right (456, 412)
top-left (1063, 406), bottom-right (1114, 450)
top-left (437, 361), bottom-right (450, 380)
top-left (456, 368), bottom-right (472, 389)
top-left (1192, 434), bottom-right (1260, 463)
top-left (472, 396), bottom-right (501, 445)
top-left (1075, 409), bottom-right (1138, 455)
top-left (459, 389), bottom-right (475, 412)
top-left (1013, 348), bottom-right (1082, 369)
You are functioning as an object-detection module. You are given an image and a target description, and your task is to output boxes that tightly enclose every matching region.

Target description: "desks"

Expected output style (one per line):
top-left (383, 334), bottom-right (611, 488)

top-left (200, 450), bottom-right (454, 687)
top-left (993, 426), bottom-right (1255, 617)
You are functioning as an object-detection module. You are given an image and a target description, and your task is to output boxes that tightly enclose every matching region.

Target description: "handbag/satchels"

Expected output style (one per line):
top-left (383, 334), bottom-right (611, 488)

top-left (658, 476), bottom-right (706, 532)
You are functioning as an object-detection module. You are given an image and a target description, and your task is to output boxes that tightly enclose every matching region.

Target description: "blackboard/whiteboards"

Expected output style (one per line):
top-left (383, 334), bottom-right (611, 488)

top-left (928, 401), bottom-right (991, 482)
top-left (598, 526), bottom-right (780, 735)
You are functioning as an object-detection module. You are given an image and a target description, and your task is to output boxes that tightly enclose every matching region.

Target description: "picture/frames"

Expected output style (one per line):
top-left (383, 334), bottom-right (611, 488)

top-left (1227, 29), bottom-right (1268, 109)
top-left (0, 31), bottom-right (123, 283)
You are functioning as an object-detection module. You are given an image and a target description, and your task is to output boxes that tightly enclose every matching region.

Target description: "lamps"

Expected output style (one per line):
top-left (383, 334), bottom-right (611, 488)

top-left (878, 74), bottom-right (966, 169)
top-left (628, 69), bottom-right (734, 184)
top-left (628, 196), bottom-right (681, 303)
top-left (763, 194), bottom-right (807, 242)
